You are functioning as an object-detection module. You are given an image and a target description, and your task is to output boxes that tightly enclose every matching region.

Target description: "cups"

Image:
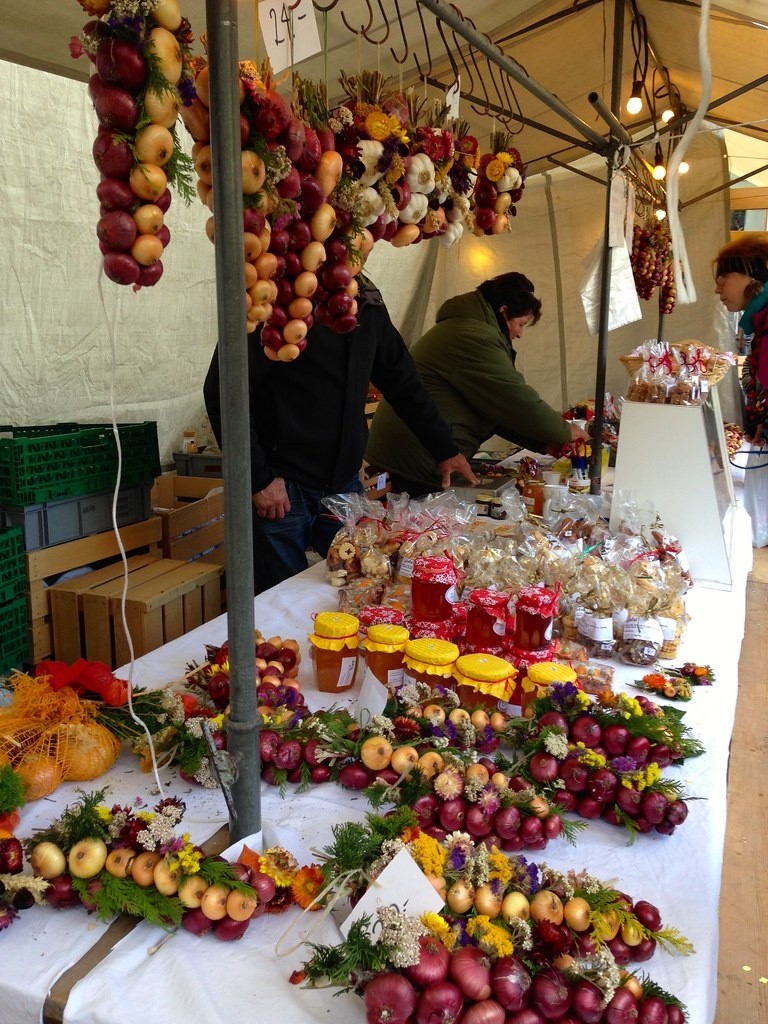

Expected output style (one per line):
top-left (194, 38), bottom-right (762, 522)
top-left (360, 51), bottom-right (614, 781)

top-left (572, 456), bottom-right (587, 478)
top-left (541, 470), bottom-right (562, 484)
top-left (543, 485), bottom-right (569, 501)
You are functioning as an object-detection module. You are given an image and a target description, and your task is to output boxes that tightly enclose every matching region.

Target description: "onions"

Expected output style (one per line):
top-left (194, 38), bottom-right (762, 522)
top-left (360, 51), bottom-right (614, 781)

top-left (0, 0), bottom-right (708, 1024)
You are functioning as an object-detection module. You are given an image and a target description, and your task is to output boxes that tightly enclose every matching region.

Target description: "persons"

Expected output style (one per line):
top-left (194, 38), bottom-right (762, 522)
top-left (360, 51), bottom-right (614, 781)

top-left (714, 231), bottom-right (768, 448)
top-left (204, 268), bottom-right (480, 593)
top-left (365, 272), bottom-right (591, 501)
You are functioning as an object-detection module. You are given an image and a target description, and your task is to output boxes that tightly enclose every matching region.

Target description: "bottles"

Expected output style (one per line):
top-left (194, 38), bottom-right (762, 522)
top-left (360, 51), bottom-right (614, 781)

top-left (306, 556), bottom-right (577, 721)
top-left (473, 477), bottom-right (546, 521)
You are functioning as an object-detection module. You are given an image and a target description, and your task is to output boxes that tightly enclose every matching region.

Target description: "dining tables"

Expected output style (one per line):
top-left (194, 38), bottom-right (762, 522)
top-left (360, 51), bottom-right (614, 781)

top-left (4, 392), bottom-right (768, 1023)
